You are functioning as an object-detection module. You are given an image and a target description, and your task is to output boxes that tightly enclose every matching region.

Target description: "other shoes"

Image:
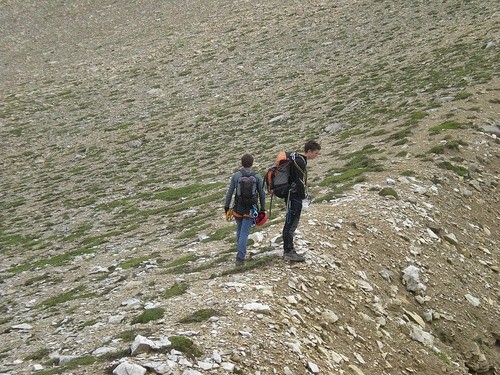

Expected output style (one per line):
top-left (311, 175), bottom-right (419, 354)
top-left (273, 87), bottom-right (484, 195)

top-left (284, 251), bottom-right (305, 262)
top-left (235, 259), bottom-right (245, 268)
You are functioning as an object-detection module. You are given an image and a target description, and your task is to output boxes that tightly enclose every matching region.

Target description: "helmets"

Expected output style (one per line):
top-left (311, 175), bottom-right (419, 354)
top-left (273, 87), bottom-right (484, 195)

top-left (255, 211), bottom-right (267, 225)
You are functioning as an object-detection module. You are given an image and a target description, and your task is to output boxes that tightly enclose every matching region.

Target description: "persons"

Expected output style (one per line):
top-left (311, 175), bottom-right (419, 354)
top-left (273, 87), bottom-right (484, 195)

top-left (225, 154), bottom-right (265, 264)
top-left (283, 141), bottom-right (321, 261)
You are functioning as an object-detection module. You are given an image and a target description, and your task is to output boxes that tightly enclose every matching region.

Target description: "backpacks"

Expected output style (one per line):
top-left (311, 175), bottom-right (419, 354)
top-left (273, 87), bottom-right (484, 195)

top-left (235, 169), bottom-right (258, 203)
top-left (266, 151), bottom-right (307, 199)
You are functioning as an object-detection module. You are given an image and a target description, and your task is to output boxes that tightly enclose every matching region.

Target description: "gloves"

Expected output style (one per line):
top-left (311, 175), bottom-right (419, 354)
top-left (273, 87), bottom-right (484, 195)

top-left (225, 210), bottom-right (232, 222)
top-left (301, 199), bottom-right (308, 213)
